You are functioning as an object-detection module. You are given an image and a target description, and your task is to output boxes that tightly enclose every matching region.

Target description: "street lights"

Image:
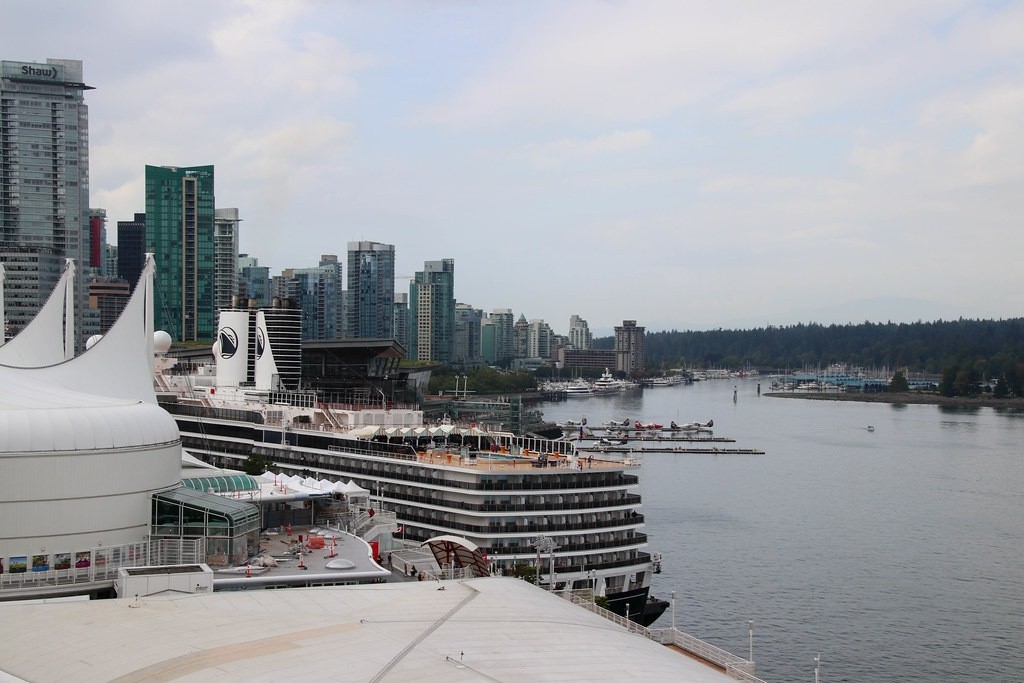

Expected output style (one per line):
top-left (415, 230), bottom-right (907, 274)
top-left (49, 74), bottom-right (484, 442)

top-left (749, 618), bottom-right (754, 662)
top-left (814, 652), bottom-right (821, 683)
top-left (454, 375), bottom-right (459, 397)
top-left (463, 376), bottom-right (468, 400)
top-left (626, 603), bottom-right (630, 630)
top-left (672, 590), bottom-right (676, 627)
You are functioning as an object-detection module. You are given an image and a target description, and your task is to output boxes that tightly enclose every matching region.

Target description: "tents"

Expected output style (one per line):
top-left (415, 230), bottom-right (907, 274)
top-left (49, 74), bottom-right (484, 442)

top-left (260, 470), bottom-right (372, 515)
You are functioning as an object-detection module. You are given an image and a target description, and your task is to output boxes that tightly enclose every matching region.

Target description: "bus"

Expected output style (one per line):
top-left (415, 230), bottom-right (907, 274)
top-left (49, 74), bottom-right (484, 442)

top-left (444, 390), bottom-right (477, 396)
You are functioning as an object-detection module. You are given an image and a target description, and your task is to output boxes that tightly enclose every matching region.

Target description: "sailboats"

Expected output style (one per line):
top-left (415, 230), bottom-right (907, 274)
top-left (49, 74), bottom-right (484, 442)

top-left (691, 358), bottom-right (757, 381)
top-left (771, 361), bottom-right (943, 390)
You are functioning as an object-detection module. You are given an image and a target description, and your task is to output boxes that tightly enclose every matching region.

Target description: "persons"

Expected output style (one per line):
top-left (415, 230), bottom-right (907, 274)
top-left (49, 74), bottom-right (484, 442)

top-left (588, 456), bottom-right (593, 469)
top-left (410, 565), bottom-right (426, 581)
top-left (537, 451), bottom-right (548, 461)
top-left (387, 552), bottom-right (394, 571)
top-left (490, 443), bottom-right (512, 454)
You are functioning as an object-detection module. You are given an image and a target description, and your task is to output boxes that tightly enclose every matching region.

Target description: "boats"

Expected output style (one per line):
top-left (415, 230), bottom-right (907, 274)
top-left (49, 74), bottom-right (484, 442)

top-left (544, 377), bottom-right (593, 395)
top-left (593, 368), bottom-right (623, 397)
top-left (625, 369), bottom-right (688, 389)
top-left (161, 387), bottom-right (670, 629)
